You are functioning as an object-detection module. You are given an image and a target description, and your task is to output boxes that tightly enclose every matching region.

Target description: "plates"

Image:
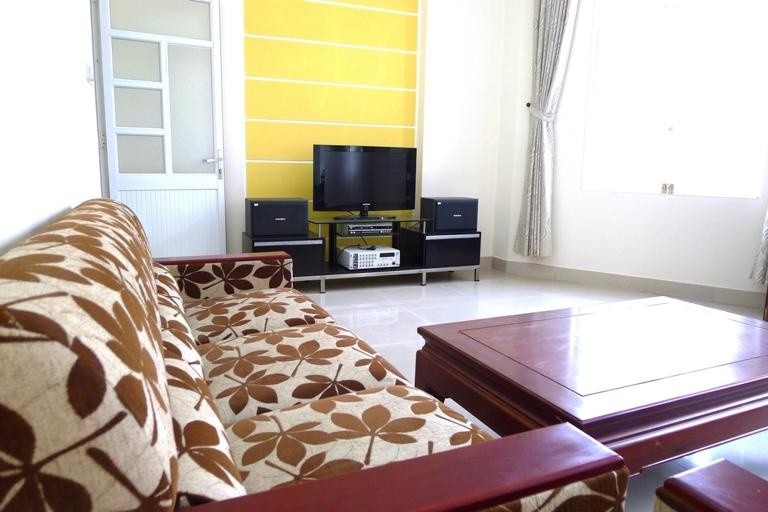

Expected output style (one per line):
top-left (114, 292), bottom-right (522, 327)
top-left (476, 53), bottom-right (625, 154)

top-left (241, 216), bottom-right (483, 294)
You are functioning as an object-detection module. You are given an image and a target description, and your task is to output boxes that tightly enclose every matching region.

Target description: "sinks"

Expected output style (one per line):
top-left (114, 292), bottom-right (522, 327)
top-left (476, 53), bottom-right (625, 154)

top-left (1, 200), bottom-right (627, 507)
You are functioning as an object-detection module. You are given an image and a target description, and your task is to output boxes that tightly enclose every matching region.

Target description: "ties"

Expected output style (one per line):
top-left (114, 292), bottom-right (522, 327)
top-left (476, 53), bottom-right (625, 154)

top-left (313, 145), bottom-right (417, 219)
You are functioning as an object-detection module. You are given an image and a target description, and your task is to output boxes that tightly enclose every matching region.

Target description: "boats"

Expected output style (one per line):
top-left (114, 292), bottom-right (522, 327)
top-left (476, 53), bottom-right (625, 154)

top-left (245, 198), bottom-right (308, 238)
top-left (420, 197), bottom-right (478, 234)
